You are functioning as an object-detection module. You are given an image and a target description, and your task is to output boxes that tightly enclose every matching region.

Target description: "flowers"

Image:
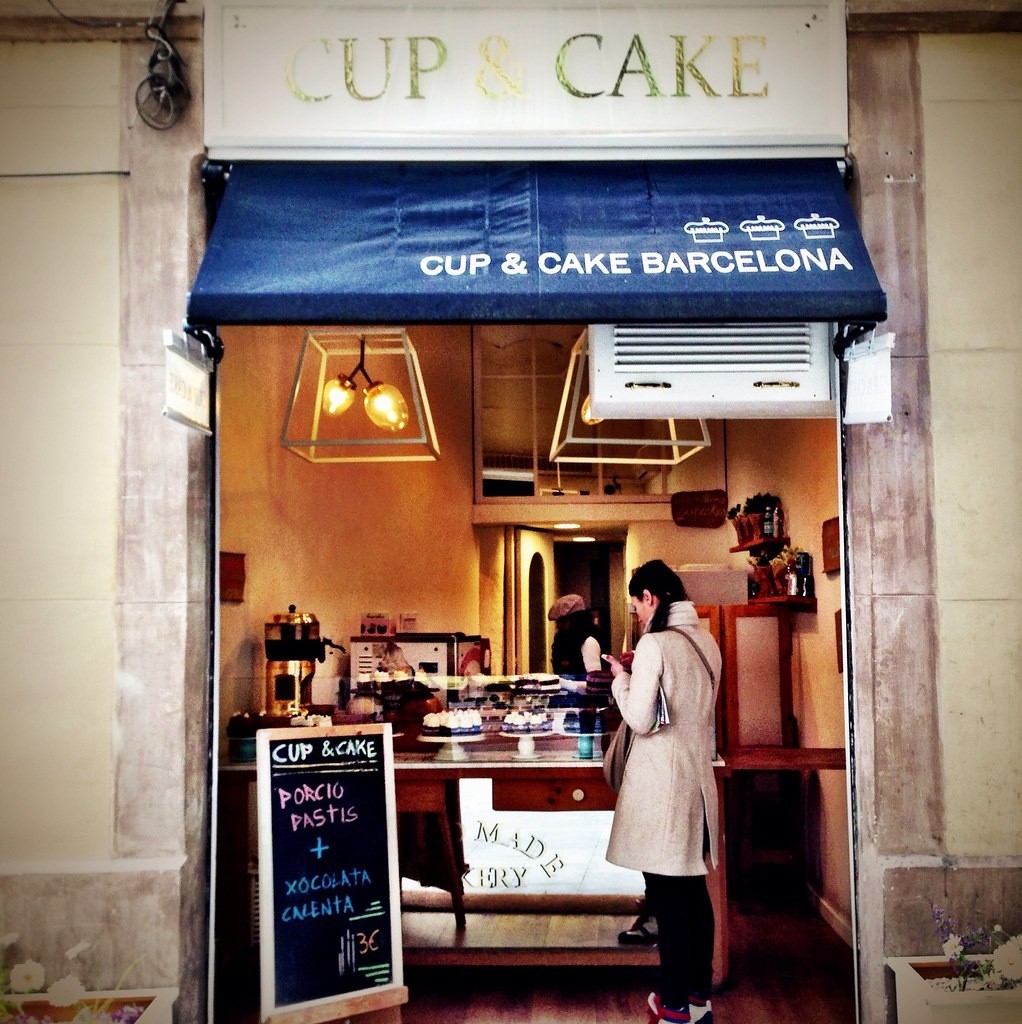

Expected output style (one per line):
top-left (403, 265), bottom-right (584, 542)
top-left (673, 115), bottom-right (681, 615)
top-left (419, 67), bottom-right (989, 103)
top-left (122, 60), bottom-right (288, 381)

top-left (744, 544), bottom-right (805, 573)
top-left (0, 932), bottom-right (146, 1024)
top-left (930, 903), bottom-right (1022, 992)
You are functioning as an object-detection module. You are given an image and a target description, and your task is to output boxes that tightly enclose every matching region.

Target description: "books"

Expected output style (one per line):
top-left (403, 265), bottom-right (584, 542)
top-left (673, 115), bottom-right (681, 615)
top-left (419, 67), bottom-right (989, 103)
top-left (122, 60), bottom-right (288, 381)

top-left (644, 683), bottom-right (671, 737)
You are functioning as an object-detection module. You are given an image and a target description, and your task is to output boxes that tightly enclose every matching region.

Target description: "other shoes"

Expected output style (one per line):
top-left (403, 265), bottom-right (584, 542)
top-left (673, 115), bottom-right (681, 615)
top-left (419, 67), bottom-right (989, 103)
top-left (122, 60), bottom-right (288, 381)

top-left (645, 993), bottom-right (713, 1024)
top-left (617, 913), bottom-right (660, 944)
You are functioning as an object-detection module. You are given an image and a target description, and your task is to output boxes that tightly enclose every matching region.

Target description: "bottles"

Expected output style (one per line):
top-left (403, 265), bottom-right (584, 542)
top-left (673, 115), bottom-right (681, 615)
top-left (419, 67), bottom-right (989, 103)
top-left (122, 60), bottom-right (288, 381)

top-left (763, 506), bottom-right (773, 537)
top-left (772, 506), bottom-right (784, 537)
top-left (783, 563), bottom-right (798, 595)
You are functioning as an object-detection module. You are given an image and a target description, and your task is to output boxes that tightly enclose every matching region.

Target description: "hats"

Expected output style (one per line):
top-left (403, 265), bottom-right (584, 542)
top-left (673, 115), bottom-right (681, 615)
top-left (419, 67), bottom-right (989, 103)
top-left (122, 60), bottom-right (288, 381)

top-left (548, 594), bottom-right (585, 620)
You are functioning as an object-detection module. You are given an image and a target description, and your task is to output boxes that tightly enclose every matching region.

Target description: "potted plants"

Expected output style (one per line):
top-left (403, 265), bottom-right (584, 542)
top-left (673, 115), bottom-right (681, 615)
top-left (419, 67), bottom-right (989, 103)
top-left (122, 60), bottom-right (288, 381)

top-left (726, 493), bottom-right (783, 548)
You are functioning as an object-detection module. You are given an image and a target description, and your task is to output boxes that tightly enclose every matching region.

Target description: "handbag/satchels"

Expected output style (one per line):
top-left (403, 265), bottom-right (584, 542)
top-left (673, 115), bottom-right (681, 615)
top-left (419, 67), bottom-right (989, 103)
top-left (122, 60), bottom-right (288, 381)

top-left (602, 718), bottom-right (636, 794)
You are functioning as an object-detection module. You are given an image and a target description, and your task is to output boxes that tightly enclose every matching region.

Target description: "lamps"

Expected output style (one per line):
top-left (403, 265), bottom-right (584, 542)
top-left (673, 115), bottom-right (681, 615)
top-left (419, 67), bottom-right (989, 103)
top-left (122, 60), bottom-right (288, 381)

top-left (280, 326), bottom-right (443, 465)
top-left (550, 330), bottom-right (712, 466)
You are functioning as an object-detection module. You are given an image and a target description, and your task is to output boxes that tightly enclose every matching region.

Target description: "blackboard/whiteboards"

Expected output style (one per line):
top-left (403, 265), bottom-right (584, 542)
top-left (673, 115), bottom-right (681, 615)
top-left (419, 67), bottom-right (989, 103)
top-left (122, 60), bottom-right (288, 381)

top-left (255, 720), bottom-right (410, 1024)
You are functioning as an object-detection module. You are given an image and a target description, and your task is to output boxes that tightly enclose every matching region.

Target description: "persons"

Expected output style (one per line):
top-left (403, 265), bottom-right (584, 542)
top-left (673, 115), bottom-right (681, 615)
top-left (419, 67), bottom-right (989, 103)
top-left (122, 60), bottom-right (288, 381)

top-left (606, 560), bottom-right (724, 1024)
top-left (547, 594), bottom-right (605, 675)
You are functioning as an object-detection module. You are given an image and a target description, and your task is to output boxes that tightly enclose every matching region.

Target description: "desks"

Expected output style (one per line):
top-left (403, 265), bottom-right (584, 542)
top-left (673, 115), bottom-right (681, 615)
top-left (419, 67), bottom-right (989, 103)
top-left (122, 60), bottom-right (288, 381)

top-left (220, 752), bottom-right (731, 991)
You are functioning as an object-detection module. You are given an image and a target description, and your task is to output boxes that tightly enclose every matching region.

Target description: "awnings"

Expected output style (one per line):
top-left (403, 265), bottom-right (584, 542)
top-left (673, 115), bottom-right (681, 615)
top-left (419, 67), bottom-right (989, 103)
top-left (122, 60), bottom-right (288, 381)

top-left (187, 162), bottom-right (888, 359)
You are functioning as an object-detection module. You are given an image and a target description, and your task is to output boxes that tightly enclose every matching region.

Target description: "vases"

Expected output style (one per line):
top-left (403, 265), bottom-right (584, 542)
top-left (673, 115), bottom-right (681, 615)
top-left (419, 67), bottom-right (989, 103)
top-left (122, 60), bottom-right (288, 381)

top-left (0, 986), bottom-right (181, 1024)
top-left (751, 567), bottom-right (787, 597)
top-left (887, 954), bottom-right (1022, 1024)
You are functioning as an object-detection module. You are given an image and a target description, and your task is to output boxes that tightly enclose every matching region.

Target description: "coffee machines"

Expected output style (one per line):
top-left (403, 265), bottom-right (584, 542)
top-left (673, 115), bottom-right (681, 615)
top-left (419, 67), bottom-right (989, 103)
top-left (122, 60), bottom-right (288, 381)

top-left (263, 604), bottom-right (346, 719)
